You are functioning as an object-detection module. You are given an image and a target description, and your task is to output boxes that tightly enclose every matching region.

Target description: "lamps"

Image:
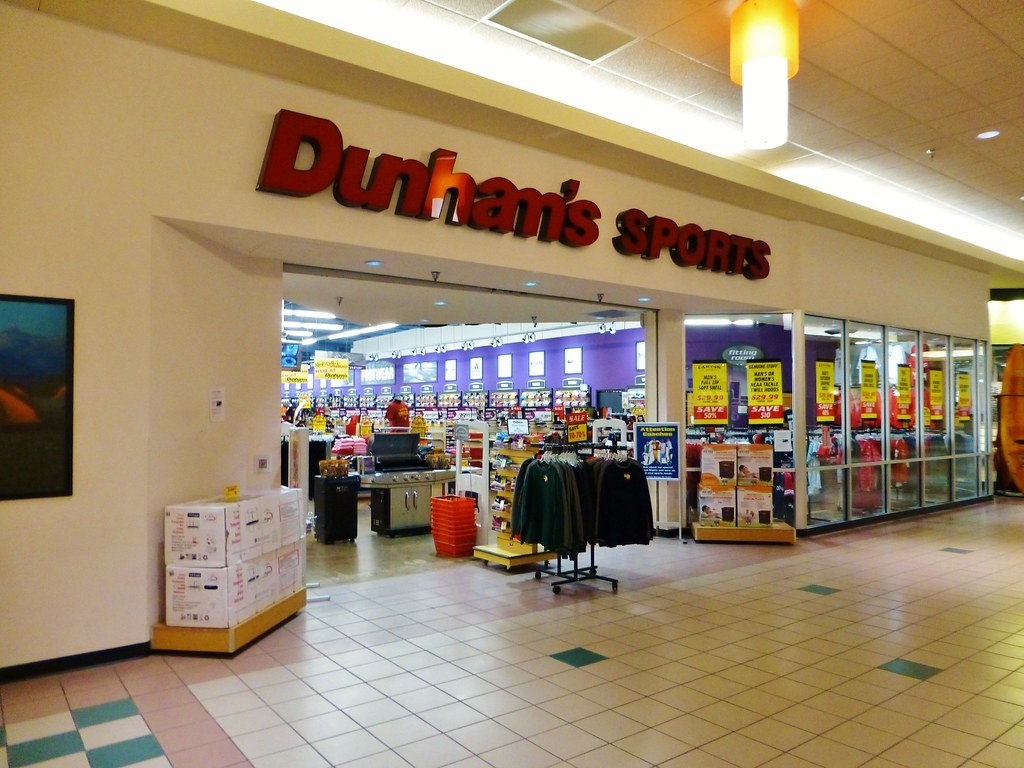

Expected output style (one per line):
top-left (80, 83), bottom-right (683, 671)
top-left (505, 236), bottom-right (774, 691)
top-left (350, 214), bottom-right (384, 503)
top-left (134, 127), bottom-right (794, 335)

top-left (369, 354), bottom-right (373, 360)
top-left (375, 353), bottom-right (379, 361)
top-left (412, 348), bottom-right (417, 355)
top-left (522, 333), bottom-right (529, 343)
top-left (599, 324), bottom-right (606, 334)
top-left (435, 345), bottom-right (440, 353)
top-left (421, 347), bottom-right (426, 355)
top-left (398, 351), bottom-right (402, 358)
top-left (442, 344), bottom-right (447, 352)
top-left (469, 340), bottom-right (474, 349)
top-left (530, 332), bottom-right (536, 342)
top-left (490, 338), bottom-right (496, 347)
top-left (497, 337), bottom-right (503, 346)
top-left (730, 0), bottom-right (800, 151)
top-left (462, 341), bottom-right (468, 350)
top-left (392, 352), bottom-right (396, 358)
top-left (609, 322), bottom-right (616, 334)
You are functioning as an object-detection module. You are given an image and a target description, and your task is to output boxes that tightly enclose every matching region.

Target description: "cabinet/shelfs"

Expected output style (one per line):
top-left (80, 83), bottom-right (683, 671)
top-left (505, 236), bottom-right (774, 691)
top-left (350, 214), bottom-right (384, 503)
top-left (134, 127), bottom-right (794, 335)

top-left (370, 486), bottom-right (431, 537)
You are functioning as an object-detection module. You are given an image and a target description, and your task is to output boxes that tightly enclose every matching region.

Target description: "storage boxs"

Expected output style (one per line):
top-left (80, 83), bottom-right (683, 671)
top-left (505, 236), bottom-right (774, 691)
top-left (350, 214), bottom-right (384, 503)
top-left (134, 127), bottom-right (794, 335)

top-left (697, 483), bottom-right (736, 527)
top-left (163, 489), bottom-right (305, 629)
top-left (701, 445), bottom-right (737, 485)
top-left (736, 488), bottom-right (773, 527)
top-left (737, 444), bottom-right (773, 487)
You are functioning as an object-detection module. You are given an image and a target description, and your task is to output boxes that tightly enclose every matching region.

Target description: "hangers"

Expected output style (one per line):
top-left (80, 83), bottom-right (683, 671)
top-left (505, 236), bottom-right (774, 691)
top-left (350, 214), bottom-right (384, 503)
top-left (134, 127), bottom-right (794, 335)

top-left (686, 427), bottom-right (942, 444)
top-left (281, 434), bottom-right (334, 444)
top-left (540, 442), bottom-right (629, 464)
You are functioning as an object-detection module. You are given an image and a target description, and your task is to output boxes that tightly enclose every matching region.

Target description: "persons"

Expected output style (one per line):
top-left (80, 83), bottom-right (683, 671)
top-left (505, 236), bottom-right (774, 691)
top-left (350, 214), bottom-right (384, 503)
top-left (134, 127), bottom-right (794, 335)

top-left (385, 396), bottom-right (409, 433)
